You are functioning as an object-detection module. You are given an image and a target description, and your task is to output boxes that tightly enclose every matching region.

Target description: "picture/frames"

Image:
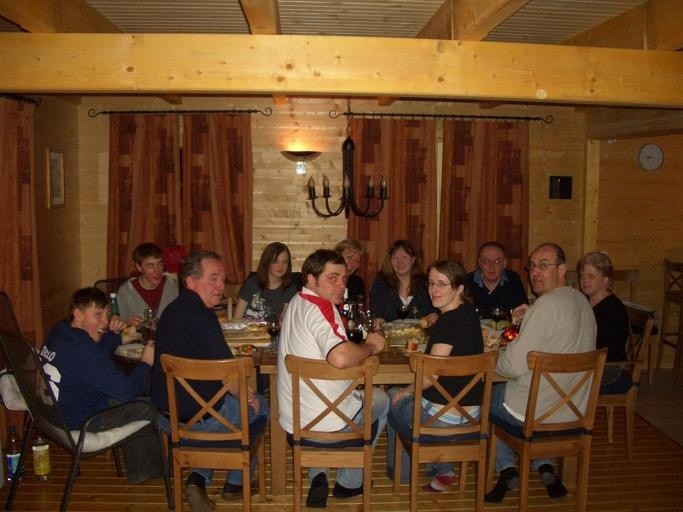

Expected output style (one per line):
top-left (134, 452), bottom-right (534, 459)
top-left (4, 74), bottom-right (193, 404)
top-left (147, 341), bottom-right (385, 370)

top-left (43, 145), bottom-right (66, 209)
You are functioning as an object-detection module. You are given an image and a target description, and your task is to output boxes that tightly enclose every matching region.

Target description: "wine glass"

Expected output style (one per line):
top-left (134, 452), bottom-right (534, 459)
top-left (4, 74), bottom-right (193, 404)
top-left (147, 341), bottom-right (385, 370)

top-left (473, 304), bottom-right (516, 329)
top-left (265, 316), bottom-right (282, 352)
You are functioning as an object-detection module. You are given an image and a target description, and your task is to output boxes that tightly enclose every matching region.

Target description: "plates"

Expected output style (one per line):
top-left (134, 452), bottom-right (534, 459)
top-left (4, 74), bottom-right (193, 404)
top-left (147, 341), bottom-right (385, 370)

top-left (247, 321), bottom-right (267, 330)
top-left (114, 343), bottom-right (143, 358)
top-left (388, 343), bottom-right (407, 348)
top-left (119, 332), bottom-right (142, 343)
top-left (401, 344), bottom-right (426, 357)
top-left (385, 317), bottom-right (420, 331)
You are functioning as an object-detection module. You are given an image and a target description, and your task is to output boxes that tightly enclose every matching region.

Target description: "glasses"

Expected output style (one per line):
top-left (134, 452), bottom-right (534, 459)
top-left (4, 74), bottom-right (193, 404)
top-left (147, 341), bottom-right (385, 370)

top-left (425, 281), bottom-right (449, 287)
top-left (525, 263), bottom-right (561, 270)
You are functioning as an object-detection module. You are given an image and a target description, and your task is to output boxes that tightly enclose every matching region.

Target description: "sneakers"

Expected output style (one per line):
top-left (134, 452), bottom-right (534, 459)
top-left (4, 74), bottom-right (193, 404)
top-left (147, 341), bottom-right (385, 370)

top-left (184, 473), bottom-right (214, 512)
top-left (307, 474), bottom-right (373, 508)
top-left (223, 480), bottom-right (259, 500)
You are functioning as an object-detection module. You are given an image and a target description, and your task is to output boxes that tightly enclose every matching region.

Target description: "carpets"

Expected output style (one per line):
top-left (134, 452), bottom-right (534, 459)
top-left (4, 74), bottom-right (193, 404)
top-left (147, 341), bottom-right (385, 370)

top-left (620, 367), bottom-right (683, 448)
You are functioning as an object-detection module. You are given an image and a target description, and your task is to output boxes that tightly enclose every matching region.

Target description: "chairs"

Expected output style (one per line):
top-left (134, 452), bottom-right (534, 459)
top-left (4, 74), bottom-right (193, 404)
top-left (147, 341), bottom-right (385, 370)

top-left (611, 268), bottom-right (662, 386)
top-left (2, 288), bottom-right (44, 444)
top-left (590, 303), bottom-right (657, 463)
top-left (157, 352), bottom-right (266, 511)
top-left (484, 345), bottom-right (610, 512)
top-left (281, 352), bottom-right (382, 511)
top-left (391, 349), bottom-right (501, 512)
top-left (0, 327), bottom-right (174, 512)
top-left (93, 277), bottom-right (130, 296)
top-left (656, 258), bottom-right (683, 388)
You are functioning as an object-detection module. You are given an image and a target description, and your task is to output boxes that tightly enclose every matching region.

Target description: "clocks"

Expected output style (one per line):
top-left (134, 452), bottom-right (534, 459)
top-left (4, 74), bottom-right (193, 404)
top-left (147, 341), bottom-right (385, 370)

top-left (636, 141), bottom-right (665, 172)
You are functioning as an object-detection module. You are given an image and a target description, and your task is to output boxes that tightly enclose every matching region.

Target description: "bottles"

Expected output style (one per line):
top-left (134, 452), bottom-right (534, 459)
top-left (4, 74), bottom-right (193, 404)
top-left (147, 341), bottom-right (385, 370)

top-left (108, 292), bottom-right (120, 317)
top-left (339, 287), bottom-right (372, 328)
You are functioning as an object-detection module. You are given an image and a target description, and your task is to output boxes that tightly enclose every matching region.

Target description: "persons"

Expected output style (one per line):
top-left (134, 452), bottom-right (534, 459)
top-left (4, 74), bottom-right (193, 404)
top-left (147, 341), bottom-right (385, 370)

top-left (231, 240), bottom-right (299, 390)
top-left (276, 248), bottom-right (391, 507)
top-left (30, 287), bottom-right (172, 487)
top-left (332, 238), bottom-right (367, 322)
top-left (574, 250), bottom-right (633, 396)
top-left (149, 247), bottom-right (269, 512)
top-left (464, 241), bottom-right (530, 331)
top-left (478, 242), bottom-right (599, 505)
top-left (112, 240), bottom-right (180, 340)
top-left (382, 259), bottom-right (488, 496)
top-left (369, 239), bottom-right (440, 329)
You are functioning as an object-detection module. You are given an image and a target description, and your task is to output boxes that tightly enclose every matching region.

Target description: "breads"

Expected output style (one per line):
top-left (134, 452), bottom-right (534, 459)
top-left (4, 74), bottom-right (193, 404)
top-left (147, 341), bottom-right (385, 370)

top-left (405, 338), bottom-right (420, 352)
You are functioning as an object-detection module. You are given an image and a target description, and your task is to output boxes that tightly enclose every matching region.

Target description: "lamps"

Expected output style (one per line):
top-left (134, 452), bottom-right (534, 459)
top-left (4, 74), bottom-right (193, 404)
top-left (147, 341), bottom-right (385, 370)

top-left (305, 95), bottom-right (390, 219)
top-left (279, 150), bottom-right (320, 164)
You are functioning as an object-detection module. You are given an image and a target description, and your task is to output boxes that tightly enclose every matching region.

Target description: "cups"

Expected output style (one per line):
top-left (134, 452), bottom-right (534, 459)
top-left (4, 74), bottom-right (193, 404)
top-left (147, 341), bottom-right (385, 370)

top-left (379, 327), bottom-right (391, 352)
top-left (346, 327), bottom-right (364, 345)
top-left (142, 307), bottom-right (156, 322)
